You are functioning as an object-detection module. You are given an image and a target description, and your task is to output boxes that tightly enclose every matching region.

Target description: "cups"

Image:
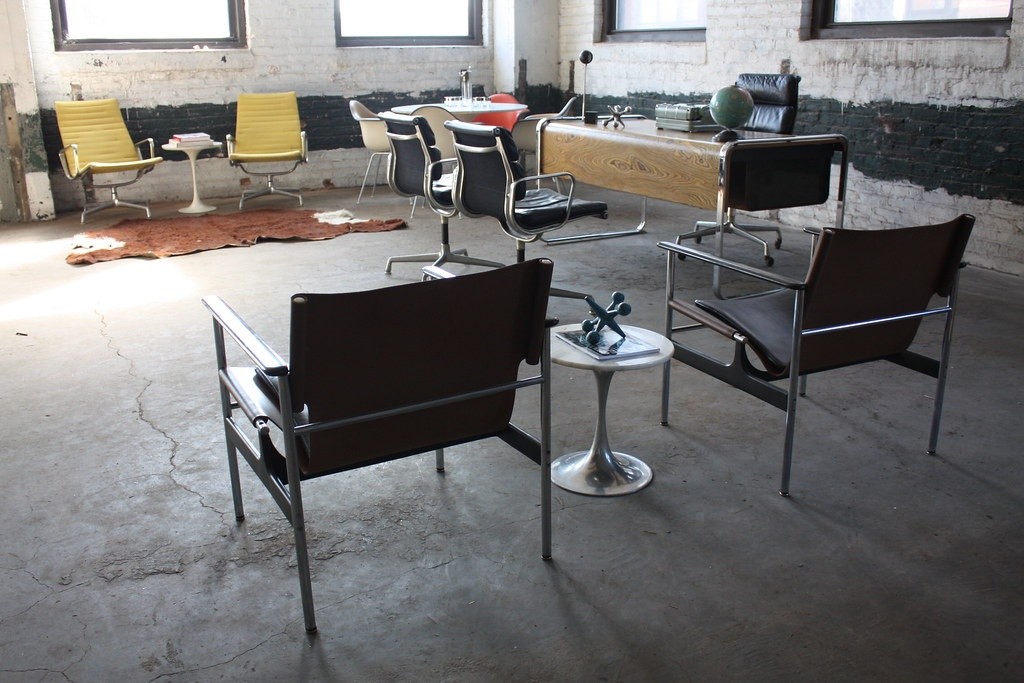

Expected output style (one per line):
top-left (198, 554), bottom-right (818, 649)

top-left (444, 96), bottom-right (486, 107)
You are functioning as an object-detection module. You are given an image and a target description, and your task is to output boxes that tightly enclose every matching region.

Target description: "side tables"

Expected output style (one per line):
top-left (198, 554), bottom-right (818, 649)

top-left (163, 141), bottom-right (223, 213)
top-left (547, 323), bottom-right (674, 497)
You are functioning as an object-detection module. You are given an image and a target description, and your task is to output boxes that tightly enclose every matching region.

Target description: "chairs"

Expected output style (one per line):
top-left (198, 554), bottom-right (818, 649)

top-left (347, 92), bottom-right (608, 329)
top-left (54, 98), bottom-right (164, 223)
top-left (658, 212), bottom-right (977, 497)
top-left (202, 257), bottom-right (555, 633)
top-left (225, 91), bottom-right (309, 210)
top-left (674, 73), bottom-right (800, 266)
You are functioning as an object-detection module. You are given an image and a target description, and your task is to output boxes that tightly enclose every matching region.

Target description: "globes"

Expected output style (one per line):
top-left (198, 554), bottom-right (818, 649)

top-left (710, 82), bottom-right (754, 143)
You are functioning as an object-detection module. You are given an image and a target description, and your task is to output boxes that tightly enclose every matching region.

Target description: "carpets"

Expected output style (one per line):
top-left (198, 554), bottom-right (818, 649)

top-left (65, 208), bottom-right (406, 265)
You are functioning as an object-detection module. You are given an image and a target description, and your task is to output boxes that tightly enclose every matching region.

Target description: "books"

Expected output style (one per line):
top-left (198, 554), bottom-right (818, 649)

top-left (168, 132), bottom-right (214, 147)
top-left (554, 326), bottom-right (660, 360)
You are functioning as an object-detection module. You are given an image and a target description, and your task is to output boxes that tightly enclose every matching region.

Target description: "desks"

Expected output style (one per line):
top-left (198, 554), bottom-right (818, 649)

top-left (391, 102), bottom-right (528, 123)
top-left (536, 113), bottom-right (849, 302)
